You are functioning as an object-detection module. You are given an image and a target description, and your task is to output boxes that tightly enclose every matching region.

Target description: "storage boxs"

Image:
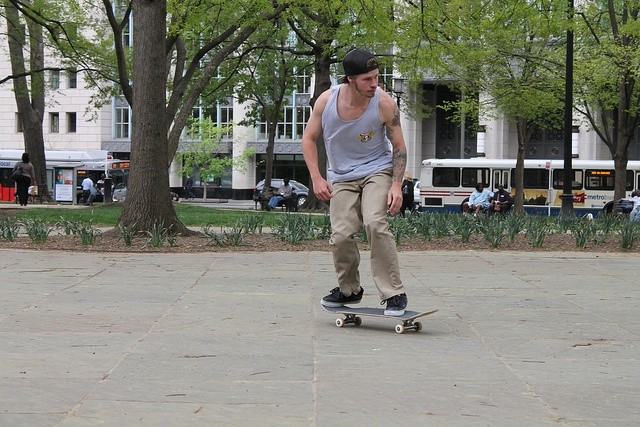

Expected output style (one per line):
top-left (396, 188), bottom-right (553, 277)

top-left (11, 184), bottom-right (46, 204)
top-left (613, 199), bottom-right (633, 219)
top-left (253, 187), bottom-right (299, 212)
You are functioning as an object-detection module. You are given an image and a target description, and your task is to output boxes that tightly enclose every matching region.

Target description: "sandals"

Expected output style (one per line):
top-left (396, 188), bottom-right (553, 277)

top-left (419, 156), bottom-right (640, 217)
top-left (0, 150), bottom-right (130, 204)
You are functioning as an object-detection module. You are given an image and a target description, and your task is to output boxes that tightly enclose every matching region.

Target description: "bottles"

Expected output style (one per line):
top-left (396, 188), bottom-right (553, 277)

top-left (342, 49), bottom-right (380, 84)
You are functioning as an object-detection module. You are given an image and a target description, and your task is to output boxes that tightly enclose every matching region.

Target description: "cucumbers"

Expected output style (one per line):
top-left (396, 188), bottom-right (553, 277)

top-left (321, 304), bottom-right (440, 334)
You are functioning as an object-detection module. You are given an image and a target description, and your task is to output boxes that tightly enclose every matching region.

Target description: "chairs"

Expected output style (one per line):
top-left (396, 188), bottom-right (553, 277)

top-left (380, 292), bottom-right (408, 316)
top-left (320, 285), bottom-right (364, 307)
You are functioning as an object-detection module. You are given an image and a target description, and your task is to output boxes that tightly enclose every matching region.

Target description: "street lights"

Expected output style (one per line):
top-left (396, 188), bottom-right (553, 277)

top-left (10, 166), bottom-right (21, 179)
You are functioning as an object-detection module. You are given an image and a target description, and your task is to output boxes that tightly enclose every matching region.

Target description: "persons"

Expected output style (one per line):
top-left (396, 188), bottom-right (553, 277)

top-left (81, 173), bottom-right (94, 207)
top-left (14, 153), bottom-right (38, 207)
top-left (267, 178), bottom-right (292, 211)
top-left (489, 184), bottom-right (513, 217)
top-left (301, 50), bottom-right (408, 316)
top-left (466, 183), bottom-right (495, 216)
top-left (400, 172), bottom-right (420, 219)
top-left (625, 189), bottom-right (640, 223)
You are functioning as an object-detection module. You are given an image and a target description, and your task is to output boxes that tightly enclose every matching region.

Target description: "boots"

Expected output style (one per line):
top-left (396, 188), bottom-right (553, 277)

top-left (254, 179), bottom-right (309, 208)
